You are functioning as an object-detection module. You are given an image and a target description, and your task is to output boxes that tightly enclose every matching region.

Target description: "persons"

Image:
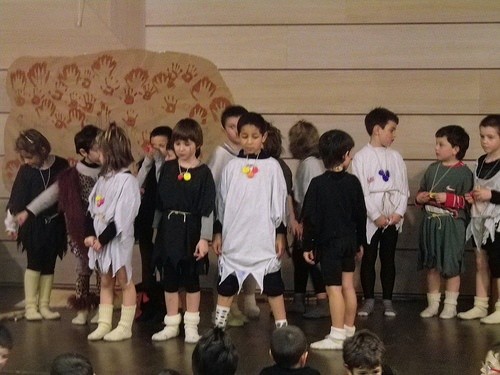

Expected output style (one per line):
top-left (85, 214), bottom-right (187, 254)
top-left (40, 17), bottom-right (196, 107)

top-left (192, 328), bottom-right (239, 375)
top-left (0, 324), bottom-right (16, 370)
top-left (4, 128), bottom-right (69, 321)
top-left (300, 130), bottom-right (368, 350)
top-left (258, 325), bottom-right (321, 375)
top-left (350, 107), bottom-right (410, 318)
top-left (81, 120), bottom-right (142, 342)
top-left (210, 103), bottom-right (332, 325)
top-left (152, 118), bottom-right (216, 344)
top-left (14, 124), bottom-right (206, 327)
top-left (50, 352), bottom-right (97, 375)
top-left (342, 328), bottom-right (393, 375)
top-left (212, 112), bottom-right (290, 331)
top-left (416, 125), bottom-right (475, 319)
top-left (458, 113), bottom-right (500, 324)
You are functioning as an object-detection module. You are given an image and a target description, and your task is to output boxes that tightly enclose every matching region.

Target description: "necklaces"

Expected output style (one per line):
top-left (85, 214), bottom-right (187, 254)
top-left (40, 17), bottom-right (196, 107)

top-left (243, 151), bottom-right (261, 177)
top-left (475, 156), bottom-right (499, 192)
top-left (366, 142), bottom-right (390, 182)
top-left (177, 158), bottom-right (196, 181)
top-left (428, 159), bottom-right (456, 199)
top-left (38, 161), bottom-right (51, 190)
